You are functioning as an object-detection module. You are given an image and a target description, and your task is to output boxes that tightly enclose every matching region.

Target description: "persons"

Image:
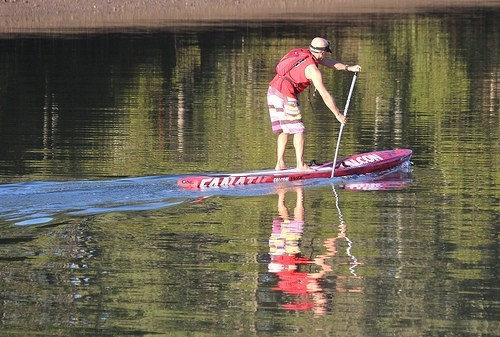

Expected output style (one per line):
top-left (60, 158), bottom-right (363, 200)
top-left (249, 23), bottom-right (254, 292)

top-left (268, 37), bottom-right (361, 174)
top-left (266, 188), bottom-right (364, 318)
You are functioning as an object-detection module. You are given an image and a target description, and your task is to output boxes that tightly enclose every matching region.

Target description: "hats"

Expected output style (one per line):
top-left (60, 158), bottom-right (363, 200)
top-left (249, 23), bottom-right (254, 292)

top-left (310, 37), bottom-right (332, 53)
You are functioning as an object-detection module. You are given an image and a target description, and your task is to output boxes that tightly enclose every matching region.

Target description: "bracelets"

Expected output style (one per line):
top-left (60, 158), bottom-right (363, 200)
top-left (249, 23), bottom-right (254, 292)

top-left (336, 111), bottom-right (341, 118)
top-left (345, 64), bottom-right (349, 71)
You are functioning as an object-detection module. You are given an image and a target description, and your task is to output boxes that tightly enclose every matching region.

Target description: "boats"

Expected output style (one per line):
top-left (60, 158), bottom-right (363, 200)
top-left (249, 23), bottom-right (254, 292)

top-left (176, 149), bottom-right (412, 189)
